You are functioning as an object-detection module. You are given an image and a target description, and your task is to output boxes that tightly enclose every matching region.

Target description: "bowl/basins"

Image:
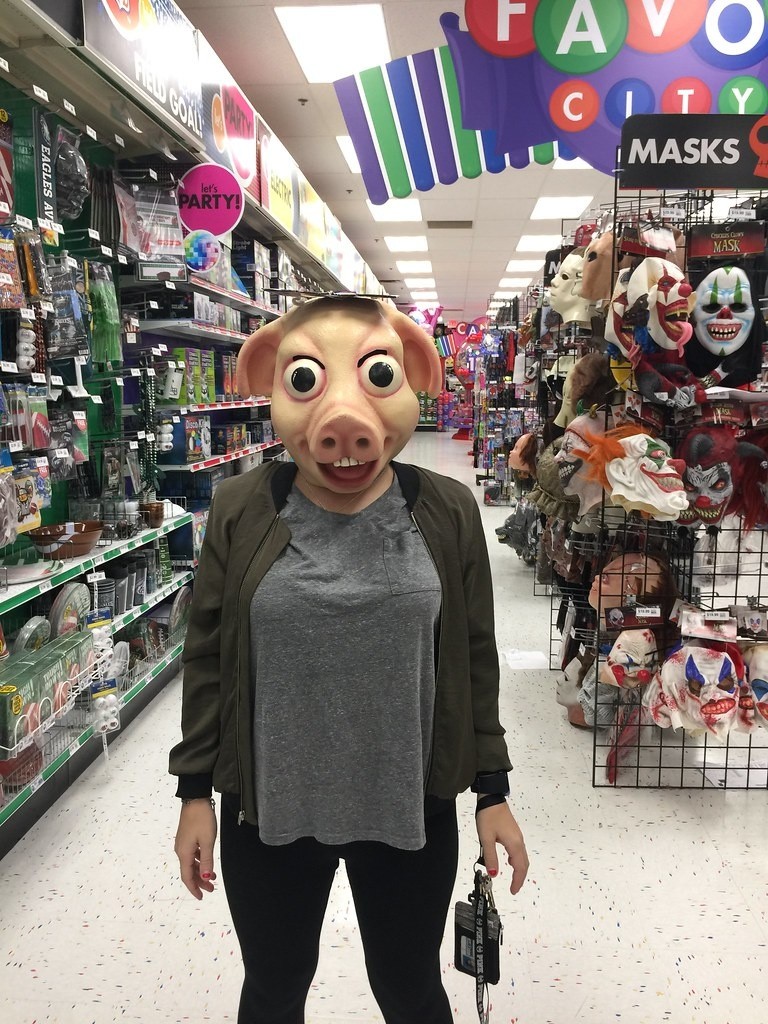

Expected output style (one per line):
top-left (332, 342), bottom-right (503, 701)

top-left (143, 503), bottom-right (164, 528)
top-left (21, 520), bottom-right (104, 560)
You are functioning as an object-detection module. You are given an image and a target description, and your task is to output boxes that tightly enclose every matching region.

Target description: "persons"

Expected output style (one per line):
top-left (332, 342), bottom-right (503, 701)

top-left (169, 295), bottom-right (529, 1024)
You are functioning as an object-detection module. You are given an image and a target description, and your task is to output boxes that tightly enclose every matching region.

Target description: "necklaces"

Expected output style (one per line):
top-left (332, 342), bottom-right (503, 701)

top-left (299, 470), bottom-right (387, 513)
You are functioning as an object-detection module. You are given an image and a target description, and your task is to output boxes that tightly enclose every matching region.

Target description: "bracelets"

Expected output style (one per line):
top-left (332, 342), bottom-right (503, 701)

top-left (181, 797), bottom-right (216, 811)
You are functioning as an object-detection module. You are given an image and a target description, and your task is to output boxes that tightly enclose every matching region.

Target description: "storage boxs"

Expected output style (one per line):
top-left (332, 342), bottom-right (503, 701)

top-left (144, 239), bottom-right (296, 561)
top-left (0, 630), bottom-right (94, 762)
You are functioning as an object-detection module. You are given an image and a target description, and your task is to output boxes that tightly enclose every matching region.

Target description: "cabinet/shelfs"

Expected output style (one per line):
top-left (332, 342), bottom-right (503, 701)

top-left (120, 0), bottom-right (335, 833)
top-left (0, 510), bottom-right (197, 854)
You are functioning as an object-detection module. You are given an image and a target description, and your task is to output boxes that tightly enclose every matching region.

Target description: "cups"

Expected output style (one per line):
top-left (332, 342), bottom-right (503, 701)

top-left (74, 537), bottom-right (172, 619)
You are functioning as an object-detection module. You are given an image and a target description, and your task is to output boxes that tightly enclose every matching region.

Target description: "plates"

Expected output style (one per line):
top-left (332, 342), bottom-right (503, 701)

top-left (0, 561), bottom-right (65, 584)
